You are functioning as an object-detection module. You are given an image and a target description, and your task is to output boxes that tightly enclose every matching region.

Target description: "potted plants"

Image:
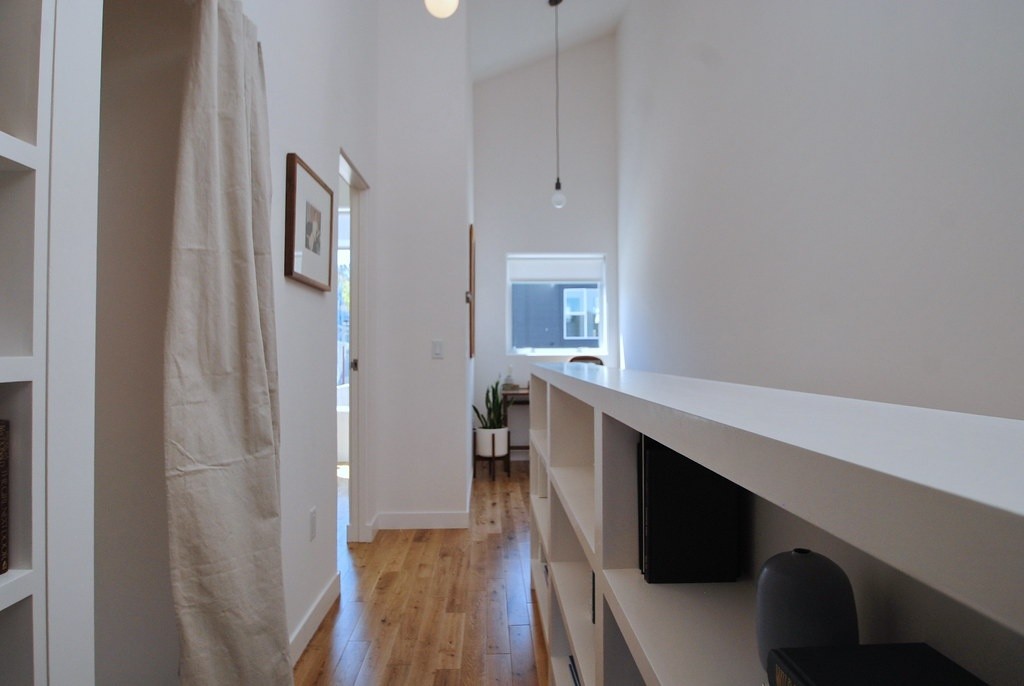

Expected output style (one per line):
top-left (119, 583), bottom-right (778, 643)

top-left (471, 380), bottom-right (511, 458)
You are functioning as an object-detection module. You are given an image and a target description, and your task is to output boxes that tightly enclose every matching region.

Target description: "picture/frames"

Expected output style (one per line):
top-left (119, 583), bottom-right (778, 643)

top-left (285, 151), bottom-right (335, 291)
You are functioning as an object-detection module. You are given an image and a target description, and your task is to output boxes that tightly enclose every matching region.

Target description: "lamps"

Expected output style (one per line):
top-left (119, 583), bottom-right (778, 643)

top-left (550, 0), bottom-right (567, 210)
top-left (424, 0), bottom-right (460, 19)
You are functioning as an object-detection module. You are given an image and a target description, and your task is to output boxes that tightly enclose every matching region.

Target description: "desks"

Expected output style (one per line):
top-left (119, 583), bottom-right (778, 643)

top-left (501, 387), bottom-right (529, 478)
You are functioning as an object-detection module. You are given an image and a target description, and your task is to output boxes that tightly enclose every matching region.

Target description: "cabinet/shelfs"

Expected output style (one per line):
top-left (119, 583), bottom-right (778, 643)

top-left (0, 0), bottom-right (98, 686)
top-left (525, 362), bottom-right (1024, 686)
